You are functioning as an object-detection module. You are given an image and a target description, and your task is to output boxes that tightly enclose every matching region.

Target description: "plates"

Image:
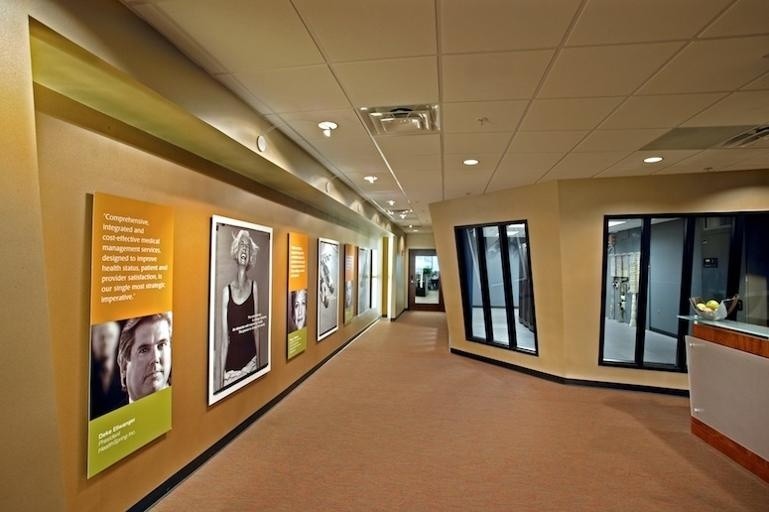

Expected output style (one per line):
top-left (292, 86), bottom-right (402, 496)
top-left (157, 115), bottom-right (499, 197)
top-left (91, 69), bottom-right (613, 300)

top-left (688, 294), bottom-right (741, 321)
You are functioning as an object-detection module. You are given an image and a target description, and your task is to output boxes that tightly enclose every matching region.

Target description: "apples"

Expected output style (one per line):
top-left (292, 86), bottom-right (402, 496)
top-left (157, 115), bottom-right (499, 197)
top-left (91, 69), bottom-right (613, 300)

top-left (696, 300), bottom-right (719, 314)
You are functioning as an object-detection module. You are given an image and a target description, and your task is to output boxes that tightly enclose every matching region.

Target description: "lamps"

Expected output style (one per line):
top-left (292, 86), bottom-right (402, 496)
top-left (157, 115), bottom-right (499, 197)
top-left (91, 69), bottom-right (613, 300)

top-left (357, 197), bottom-right (395, 212)
top-left (326, 173), bottom-right (374, 192)
top-left (256, 120), bottom-right (332, 152)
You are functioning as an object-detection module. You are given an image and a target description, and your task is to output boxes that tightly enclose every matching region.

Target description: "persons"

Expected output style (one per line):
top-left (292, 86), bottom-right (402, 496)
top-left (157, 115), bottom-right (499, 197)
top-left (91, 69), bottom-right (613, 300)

top-left (221, 229), bottom-right (261, 387)
top-left (91, 322), bottom-right (121, 418)
top-left (292, 288), bottom-right (306, 330)
top-left (116, 315), bottom-right (171, 402)
top-left (346, 281), bottom-right (352, 308)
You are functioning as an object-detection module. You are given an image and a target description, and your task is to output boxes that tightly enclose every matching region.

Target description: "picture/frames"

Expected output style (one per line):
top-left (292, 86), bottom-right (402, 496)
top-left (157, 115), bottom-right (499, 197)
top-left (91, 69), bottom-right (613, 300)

top-left (206, 215), bottom-right (272, 407)
top-left (317, 237), bottom-right (340, 341)
top-left (356, 246), bottom-right (370, 317)
